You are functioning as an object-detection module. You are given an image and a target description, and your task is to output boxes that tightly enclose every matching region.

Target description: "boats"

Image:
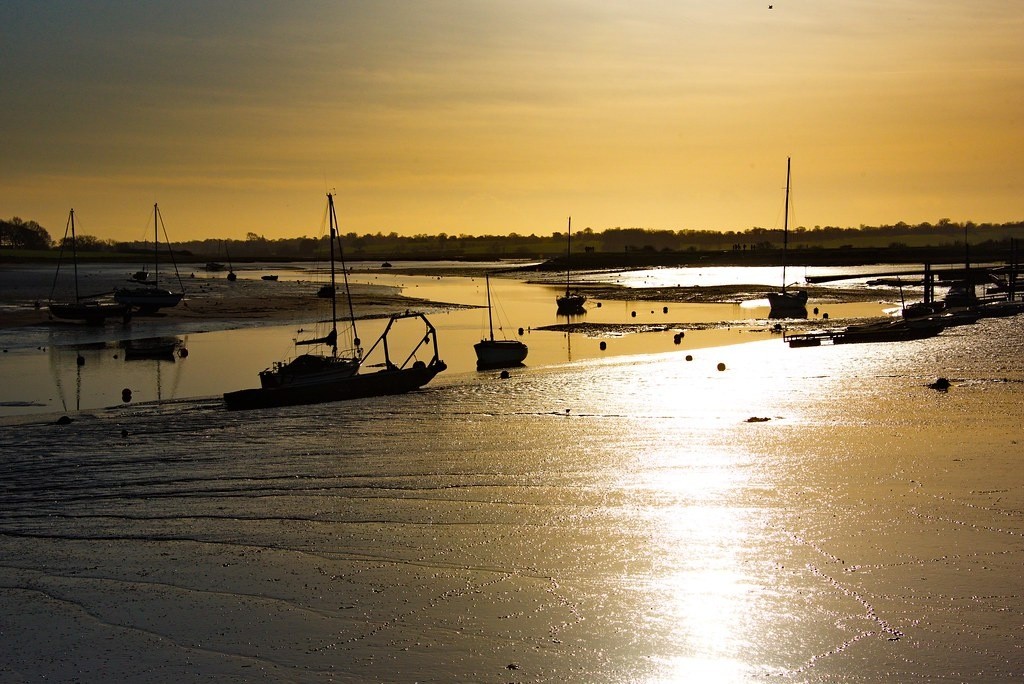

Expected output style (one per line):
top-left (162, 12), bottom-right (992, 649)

top-left (381, 259), bottom-right (392, 267)
top-left (261, 275), bottom-right (279, 280)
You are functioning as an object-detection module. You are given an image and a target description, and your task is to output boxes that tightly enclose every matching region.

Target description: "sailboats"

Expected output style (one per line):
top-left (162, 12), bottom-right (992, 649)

top-left (111, 202), bottom-right (186, 308)
top-left (473, 272), bottom-right (529, 364)
top-left (944, 224), bottom-right (1006, 305)
top-left (558, 217), bottom-right (587, 310)
top-left (42, 207), bottom-right (120, 320)
top-left (205, 239), bottom-right (225, 271)
top-left (983, 236), bottom-right (1024, 318)
top-left (257, 192), bottom-right (364, 386)
top-left (902, 255), bottom-right (980, 327)
top-left (841, 277), bottom-right (945, 339)
top-left (767, 156), bottom-right (811, 308)
